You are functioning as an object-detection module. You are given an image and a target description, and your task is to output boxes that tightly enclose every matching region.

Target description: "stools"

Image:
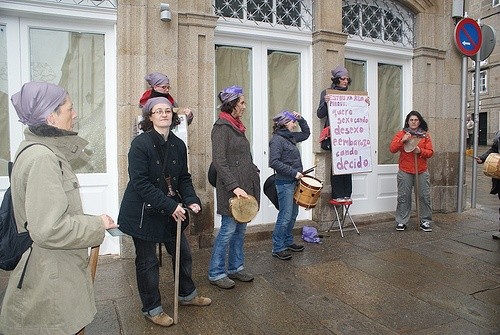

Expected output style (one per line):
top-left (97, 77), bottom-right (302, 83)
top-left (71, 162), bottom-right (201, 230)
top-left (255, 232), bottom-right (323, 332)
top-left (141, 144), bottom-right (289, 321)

top-left (328, 200), bottom-right (360, 238)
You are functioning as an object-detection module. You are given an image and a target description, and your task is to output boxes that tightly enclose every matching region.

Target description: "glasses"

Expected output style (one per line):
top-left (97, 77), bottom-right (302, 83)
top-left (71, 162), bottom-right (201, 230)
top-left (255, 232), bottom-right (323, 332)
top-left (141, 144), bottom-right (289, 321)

top-left (151, 109), bottom-right (172, 115)
top-left (158, 86), bottom-right (172, 90)
top-left (340, 76), bottom-right (350, 81)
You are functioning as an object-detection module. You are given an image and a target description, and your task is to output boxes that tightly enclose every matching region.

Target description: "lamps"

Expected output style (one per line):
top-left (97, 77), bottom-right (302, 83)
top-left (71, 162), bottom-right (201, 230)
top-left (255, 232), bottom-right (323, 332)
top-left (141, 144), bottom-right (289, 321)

top-left (160, 3), bottom-right (172, 21)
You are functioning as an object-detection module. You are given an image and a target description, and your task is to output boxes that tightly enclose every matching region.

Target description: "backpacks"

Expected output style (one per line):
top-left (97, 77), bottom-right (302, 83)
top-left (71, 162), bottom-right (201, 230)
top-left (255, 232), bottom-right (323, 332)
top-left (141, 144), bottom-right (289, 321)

top-left (0, 143), bottom-right (63, 271)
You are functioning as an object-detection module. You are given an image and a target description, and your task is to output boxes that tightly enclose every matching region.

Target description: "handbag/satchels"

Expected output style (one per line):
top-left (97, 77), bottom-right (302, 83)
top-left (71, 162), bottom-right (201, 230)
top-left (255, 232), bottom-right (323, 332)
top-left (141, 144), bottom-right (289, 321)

top-left (208, 162), bottom-right (217, 187)
top-left (321, 126), bottom-right (331, 150)
top-left (263, 170), bottom-right (280, 211)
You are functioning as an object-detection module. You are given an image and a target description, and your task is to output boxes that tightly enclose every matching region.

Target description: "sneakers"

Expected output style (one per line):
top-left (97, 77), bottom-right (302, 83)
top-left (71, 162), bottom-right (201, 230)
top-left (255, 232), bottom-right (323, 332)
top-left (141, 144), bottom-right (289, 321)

top-left (396, 223), bottom-right (407, 230)
top-left (420, 221), bottom-right (433, 232)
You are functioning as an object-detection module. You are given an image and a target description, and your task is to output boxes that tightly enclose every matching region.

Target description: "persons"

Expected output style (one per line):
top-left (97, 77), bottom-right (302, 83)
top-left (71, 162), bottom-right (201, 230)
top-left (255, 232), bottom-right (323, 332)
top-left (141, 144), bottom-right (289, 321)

top-left (317, 65), bottom-right (370, 202)
top-left (475, 131), bottom-right (500, 239)
top-left (207, 86), bottom-right (261, 290)
top-left (466, 116), bottom-right (487, 149)
top-left (139, 73), bottom-right (194, 126)
top-left (117, 97), bottom-right (211, 325)
top-left (390, 110), bottom-right (435, 231)
top-left (0, 82), bottom-right (119, 335)
top-left (268, 112), bottom-right (310, 260)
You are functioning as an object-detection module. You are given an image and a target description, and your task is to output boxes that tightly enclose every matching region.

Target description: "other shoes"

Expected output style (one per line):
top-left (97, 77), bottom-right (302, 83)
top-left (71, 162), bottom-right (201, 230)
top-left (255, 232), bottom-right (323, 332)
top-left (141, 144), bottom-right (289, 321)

top-left (492, 232), bottom-right (500, 238)
top-left (345, 198), bottom-right (352, 202)
top-left (228, 269), bottom-right (254, 282)
top-left (180, 295), bottom-right (212, 305)
top-left (287, 244), bottom-right (305, 252)
top-left (210, 276), bottom-right (235, 289)
top-left (145, 311), bottom-right (174, 327)
top-left (272, 250), bottom-right (292, 259)
top-left (333, 198), bottom-right (346, 202)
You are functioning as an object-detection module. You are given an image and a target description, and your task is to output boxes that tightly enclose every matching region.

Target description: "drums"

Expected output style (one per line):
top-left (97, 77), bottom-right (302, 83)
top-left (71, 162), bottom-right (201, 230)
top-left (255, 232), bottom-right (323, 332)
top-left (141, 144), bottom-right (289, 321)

top-left (293, 175), bottom-right (323, 209)
top-left (482, 153), bottom-right (500, 179)
top-left (232, 194), bottom-right (258, 224)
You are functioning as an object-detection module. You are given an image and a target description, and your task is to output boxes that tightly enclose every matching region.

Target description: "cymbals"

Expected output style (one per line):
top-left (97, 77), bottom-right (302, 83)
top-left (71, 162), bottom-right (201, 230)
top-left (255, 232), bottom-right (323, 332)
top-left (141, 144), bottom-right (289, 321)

top-left (229, 197), bottom-right (259, 223)
